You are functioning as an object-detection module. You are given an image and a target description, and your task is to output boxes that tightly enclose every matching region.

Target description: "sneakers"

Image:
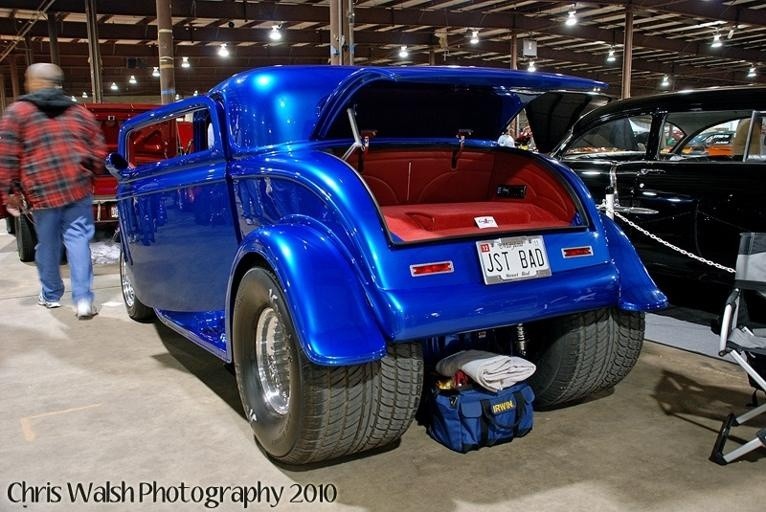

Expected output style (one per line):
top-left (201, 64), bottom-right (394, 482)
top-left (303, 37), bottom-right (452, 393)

top-left (37, 293), bottom-right (60, 308)
top-left (76, 300), bottom-right (98, 318)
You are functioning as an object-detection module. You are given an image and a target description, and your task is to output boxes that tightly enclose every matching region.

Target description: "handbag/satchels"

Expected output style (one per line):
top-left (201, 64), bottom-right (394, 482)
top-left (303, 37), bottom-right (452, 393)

top-left (427, 384), bottom-right (535, 455)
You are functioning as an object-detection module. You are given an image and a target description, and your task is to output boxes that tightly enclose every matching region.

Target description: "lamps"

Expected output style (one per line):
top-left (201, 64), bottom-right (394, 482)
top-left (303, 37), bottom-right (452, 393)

top-left (81, 6), bottom-right (757, 103)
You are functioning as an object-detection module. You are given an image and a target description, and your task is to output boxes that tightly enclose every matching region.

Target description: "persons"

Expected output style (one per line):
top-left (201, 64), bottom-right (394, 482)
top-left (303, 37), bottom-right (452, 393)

top-left (1, 63), bottom-right (109, 318)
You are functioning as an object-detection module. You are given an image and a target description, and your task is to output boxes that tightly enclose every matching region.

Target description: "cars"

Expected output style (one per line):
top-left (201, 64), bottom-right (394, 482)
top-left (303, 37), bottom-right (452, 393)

top-left (102, 63), bottom-right (671, 468)
top-left (3, 100), bottom-right (192, 262)
top-left (548, 81), bottom-right (766, 302)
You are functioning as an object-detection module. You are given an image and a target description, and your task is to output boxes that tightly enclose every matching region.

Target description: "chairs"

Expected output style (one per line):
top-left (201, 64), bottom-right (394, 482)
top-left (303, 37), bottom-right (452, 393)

top-left (709, 232), bottom-right (765, 465)
top-left (731, 118), bottom-right (763, 155)
